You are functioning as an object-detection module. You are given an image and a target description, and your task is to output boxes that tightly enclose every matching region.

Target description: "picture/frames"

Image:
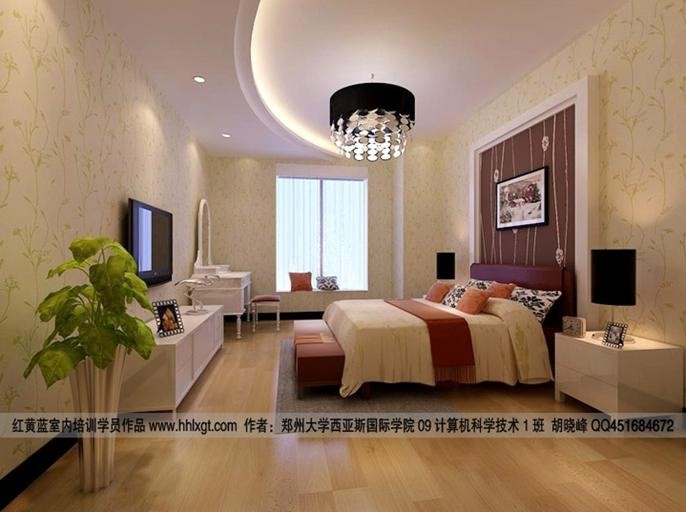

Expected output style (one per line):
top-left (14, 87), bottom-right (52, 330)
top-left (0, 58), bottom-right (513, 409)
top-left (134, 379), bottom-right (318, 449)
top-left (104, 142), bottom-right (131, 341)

top-left (151, 298), bottom-right (184, 337)
top-left (603, 322), bottom-right (628, 349)
top-left (496, 165), bottom-right (548, 231)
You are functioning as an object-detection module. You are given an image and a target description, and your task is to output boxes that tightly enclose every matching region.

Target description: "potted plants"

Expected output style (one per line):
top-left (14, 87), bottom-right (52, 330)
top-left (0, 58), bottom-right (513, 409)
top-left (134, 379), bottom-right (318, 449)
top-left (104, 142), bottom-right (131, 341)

top-left (24, 237), bottom-right (155, 493)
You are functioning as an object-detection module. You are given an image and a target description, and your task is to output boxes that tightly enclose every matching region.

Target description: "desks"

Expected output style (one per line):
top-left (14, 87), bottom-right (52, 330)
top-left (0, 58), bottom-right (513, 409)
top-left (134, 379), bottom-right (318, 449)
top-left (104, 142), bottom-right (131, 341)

top-left (190, 272), bottom-right (251, 339)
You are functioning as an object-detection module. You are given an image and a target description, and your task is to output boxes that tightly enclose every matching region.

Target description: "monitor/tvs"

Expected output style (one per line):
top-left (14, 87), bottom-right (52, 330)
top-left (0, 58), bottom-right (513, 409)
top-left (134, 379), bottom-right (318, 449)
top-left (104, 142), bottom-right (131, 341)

top-left (127, 198), bottom-right (173, 287)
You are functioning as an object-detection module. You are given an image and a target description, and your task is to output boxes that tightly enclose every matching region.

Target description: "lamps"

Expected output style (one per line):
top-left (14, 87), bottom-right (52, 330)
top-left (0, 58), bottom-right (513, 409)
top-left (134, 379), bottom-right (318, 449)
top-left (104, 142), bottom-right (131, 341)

top-left (591, 249), bottom-right (636, 343)
top-left (436, 252), bottom-right (455, 280)
top-left (330, 74), bottom-right (415, 162)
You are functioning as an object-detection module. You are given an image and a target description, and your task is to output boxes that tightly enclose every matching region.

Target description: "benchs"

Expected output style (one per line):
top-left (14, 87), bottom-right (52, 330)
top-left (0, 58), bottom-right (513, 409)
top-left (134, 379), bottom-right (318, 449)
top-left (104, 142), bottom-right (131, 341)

top-left (293, 320), bottom-right (345, 400)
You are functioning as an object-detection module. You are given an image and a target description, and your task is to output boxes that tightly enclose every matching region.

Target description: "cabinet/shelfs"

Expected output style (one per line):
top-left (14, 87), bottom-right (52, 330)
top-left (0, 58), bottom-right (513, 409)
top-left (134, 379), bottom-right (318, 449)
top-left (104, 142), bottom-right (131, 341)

top-left (118, 305), bottom-right (224, 420)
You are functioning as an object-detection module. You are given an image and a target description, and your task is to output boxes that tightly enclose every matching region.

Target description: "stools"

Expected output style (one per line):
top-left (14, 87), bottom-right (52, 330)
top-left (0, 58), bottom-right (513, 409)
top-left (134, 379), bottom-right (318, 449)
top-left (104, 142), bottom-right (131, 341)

top-left (251, 295), bottom-right (281, 332)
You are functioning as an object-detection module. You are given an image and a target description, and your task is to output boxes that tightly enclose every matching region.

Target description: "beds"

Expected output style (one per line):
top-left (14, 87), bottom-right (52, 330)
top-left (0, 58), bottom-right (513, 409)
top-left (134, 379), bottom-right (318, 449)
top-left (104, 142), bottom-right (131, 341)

top-left (322, 263), bottom-right (573, 401)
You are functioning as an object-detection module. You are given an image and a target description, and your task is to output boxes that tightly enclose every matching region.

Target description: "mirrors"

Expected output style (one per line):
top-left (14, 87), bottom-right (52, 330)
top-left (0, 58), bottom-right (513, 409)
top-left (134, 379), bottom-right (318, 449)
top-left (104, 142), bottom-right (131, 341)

top-left (193, 199), bottom-right (230, 273)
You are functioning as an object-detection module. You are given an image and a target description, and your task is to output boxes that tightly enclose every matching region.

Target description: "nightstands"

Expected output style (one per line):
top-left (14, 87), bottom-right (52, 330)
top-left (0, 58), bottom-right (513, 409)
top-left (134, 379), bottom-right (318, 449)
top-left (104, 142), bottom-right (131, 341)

top-left (555, 331), bottom-right (685, 424)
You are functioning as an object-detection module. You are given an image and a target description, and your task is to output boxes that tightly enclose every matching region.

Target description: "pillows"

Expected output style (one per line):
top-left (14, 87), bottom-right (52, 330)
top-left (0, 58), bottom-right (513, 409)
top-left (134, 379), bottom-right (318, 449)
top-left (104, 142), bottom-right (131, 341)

top-left (464, 278), bottom-right (493, 291)
top-left (456, 286), bottom-right (493, 314)
top-left (289, 272), bottom-right (312, 291)
top-left (508, 283), bottom-right (562, 323)
top-left (486, 281), bottom-right (515, 299)
top-left (442, 284), bottom-right (468, 308)
top-left (426, 281), bottom-right (454, 303)
top-left (317, 276), bottom-right (339, 290)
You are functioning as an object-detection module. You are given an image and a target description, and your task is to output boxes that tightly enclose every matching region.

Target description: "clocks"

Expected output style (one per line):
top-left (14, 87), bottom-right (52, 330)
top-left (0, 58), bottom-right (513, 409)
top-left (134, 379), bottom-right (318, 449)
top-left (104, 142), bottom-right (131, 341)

top-left (563, 316), bottom-right (586, 338)
